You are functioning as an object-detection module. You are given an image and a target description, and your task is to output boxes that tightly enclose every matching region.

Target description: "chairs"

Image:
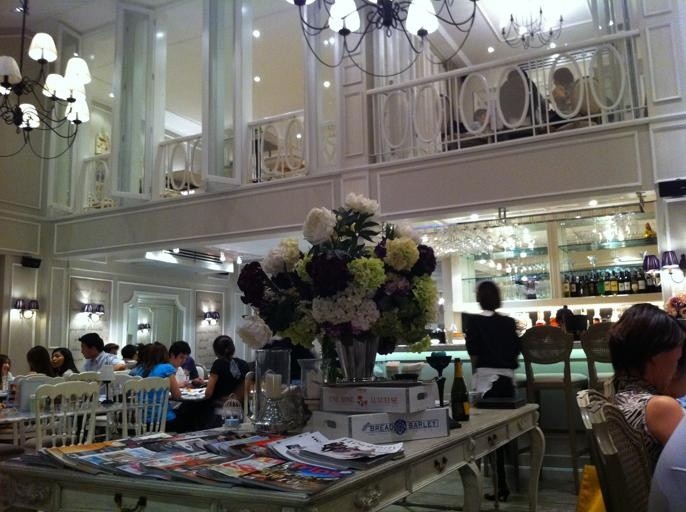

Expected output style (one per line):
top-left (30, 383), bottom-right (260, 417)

top-left (465, 317), bottom-right (659, 511)
top-left (431, 61), bottom-right (615, 152)
top-left (133, 127), bottom-right (309, 198)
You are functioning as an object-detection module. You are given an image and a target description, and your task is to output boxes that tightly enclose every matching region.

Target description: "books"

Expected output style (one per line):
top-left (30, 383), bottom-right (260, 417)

top-left (19, 427), bottom-right (407, 498)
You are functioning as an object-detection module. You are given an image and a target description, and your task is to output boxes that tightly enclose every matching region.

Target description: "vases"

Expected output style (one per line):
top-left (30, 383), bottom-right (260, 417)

top-left (330, 326), bottom-right (381, 387)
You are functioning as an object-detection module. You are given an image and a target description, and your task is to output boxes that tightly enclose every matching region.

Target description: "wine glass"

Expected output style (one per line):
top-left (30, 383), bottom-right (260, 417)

top-left (426, 354), bottom-right (453, 408)
top-left (420, 220), bottom-right (534, 259)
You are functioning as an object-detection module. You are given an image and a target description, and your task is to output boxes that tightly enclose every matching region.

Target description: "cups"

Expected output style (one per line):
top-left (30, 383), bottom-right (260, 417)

top-left (297, 357), bottom-right (324, 412)
top-left (252, 348), bottom-right (293, 432)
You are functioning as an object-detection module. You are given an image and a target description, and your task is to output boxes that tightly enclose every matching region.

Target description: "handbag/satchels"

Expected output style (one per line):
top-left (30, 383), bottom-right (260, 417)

top-left (576, 464), bottom-right (607, 512)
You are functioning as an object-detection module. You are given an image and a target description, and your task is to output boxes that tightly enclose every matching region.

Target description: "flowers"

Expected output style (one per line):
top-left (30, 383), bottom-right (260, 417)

top-left (230, 182), bottom-right (449, 386)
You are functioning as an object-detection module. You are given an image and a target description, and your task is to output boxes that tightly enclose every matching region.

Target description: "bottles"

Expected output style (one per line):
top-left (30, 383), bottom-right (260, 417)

top-left (563, 266), bottom-right (661, 297)
top-left (452, 358), bottom-right (470, 423)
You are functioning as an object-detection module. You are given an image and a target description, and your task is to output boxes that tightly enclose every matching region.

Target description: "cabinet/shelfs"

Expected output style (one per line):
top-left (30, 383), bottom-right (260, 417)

top-left (444, 200), bottom-right (665, 314)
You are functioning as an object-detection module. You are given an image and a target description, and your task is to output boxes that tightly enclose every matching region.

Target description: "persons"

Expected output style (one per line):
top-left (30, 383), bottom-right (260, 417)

top-left (0, 353), bottom-right (15, 391)
top-left (78, 331), bottom-right (207, 438)
top-left (49, 348), bottom-right (82, 379)
top-left (19, 343), bottom-right (62, 406)
top-left (201, 335), bottom-right (251, 429)
top-left (549, 66), bottom-right (579, 115)
top-left (607, 302), bottom-right (686, 510)
top-left (464, 279), bottom-right (528, 501)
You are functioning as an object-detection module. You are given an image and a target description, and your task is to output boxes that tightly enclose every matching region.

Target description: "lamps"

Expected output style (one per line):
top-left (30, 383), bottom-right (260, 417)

top-left (497, 5), bottom-right (568, 53)
top-left (80, 303), bottom-right (105, 324)
top-left (12, 295), bottom-right (40, 320)
top-left (0, 1), bottom-right (94, 163)
top-left (282, 0), bottom-right (480, 80)
top-left (137, 321), bottom-right (151, 335)
top-left (201, 310), bottom-right (221, 326)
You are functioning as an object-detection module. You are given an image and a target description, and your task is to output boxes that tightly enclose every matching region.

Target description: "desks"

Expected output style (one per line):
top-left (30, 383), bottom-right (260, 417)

top-left (1, 400), bottom-right (551, 509)
